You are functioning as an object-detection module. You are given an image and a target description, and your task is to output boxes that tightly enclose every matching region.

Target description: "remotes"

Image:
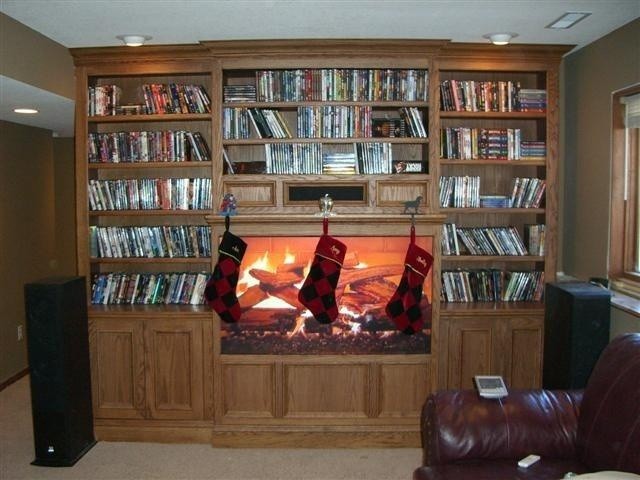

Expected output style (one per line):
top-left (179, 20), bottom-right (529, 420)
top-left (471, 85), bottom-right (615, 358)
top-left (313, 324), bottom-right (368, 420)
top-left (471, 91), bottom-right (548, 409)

top-left (518, 454), bottom-right (541, 468)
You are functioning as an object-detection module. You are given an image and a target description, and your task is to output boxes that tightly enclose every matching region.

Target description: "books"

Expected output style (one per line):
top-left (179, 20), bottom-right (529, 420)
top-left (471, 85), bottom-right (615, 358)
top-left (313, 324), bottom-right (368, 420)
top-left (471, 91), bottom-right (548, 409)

top-left (224, 67), bottom-right (428, 175)
top-left (85, 82), bottom-right (212, 306)
top-left (438, 78), bottom-right (546, 304)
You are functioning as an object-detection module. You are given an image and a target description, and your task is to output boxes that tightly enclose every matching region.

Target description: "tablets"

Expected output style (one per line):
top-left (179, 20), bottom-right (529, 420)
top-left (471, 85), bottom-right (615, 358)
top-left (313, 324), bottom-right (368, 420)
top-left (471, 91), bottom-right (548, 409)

top-left (475, 375), bottom-right (509, 397)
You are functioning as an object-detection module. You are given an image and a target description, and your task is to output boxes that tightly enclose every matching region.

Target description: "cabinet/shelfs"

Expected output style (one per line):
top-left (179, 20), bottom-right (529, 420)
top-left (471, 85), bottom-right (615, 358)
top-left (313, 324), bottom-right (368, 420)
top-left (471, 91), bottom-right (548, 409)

top-left (67, 38), bottom-right (578, 451)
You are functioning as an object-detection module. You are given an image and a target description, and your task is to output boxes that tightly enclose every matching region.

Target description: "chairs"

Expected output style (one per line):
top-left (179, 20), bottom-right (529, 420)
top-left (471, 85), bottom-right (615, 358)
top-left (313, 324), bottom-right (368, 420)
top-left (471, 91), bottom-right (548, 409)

top-left (413, 331), bottom-right (640, 479)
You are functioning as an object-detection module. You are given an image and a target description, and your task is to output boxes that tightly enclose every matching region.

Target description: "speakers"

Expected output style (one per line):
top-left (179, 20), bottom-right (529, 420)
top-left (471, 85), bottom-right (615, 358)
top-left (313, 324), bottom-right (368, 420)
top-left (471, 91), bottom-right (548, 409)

top-left (24, 275), bottom-right (98, 467)
top-left (542, 280), bottom-right (611, 390)
